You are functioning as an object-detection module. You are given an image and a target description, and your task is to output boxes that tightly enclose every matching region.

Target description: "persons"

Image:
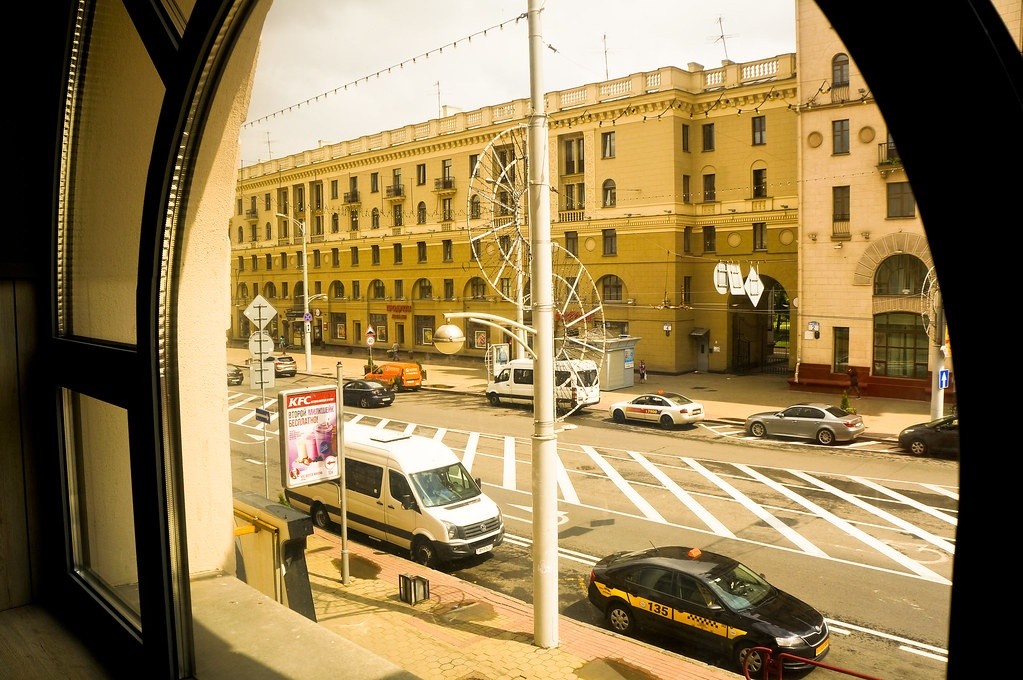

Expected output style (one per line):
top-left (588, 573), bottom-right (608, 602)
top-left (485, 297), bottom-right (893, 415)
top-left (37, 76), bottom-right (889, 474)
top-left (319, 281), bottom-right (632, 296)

top-left (392, 342), bottom-right (399, 361)
top-left (638, 360), bottom-right (646, 383)
top-left (279, 336), bottom-right (285, 348)
top-left (846, 367), bottom-right (860, 398)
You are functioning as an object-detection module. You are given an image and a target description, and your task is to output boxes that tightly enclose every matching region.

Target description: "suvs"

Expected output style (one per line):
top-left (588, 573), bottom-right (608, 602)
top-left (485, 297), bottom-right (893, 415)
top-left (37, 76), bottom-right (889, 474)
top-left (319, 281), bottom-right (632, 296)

top-left (265, 355), bottom-right (297, 378)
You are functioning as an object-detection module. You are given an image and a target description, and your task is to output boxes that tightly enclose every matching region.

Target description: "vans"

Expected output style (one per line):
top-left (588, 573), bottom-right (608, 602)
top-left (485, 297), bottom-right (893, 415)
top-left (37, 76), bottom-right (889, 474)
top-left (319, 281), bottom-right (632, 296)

top-left (364, 361), bottom-right (422, 393)
top-left (485, 358), bottom-right (600, 412)
top-left (285, 422), bottom-right (505, 571)
top-left (899, 412), bottom-right (960, 460)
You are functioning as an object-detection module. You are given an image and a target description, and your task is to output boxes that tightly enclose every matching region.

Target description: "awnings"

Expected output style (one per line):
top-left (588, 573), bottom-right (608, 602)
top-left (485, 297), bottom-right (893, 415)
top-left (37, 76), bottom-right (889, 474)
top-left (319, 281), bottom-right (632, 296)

top-left (689, 328), bottom-right (709, 335)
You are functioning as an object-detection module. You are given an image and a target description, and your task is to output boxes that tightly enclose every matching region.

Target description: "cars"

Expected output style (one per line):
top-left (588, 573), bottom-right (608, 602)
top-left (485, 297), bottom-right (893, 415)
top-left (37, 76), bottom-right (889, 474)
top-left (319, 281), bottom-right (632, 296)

top-left (588, 545), bottom-right (830, 680)
top-left (743, 402), bottom-right (866, 445)
top-left (610, 392), bottom-right (705, 428)
top-left (343, 381), bottom-right (396, 409)
top-left (226, 363), bottom-right (245, 386)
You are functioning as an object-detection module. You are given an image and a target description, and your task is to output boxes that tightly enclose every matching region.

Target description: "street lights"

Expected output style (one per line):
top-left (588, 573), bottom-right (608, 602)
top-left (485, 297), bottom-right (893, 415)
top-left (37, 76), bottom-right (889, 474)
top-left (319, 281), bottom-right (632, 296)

top-left (484, 177), bottom-right (529, 358)
top-left (433, 312), bottom-right (558, 648)
top-left (276, 212), bottom-right (329, 373)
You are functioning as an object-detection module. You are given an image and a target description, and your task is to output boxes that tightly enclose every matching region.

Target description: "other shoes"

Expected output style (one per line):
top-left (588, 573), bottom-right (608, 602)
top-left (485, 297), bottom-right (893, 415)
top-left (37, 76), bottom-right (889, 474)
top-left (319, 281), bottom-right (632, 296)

top-left (393, 358), bottom-right (400, 361)
top-left (856, 394), bottom-right (861, 398)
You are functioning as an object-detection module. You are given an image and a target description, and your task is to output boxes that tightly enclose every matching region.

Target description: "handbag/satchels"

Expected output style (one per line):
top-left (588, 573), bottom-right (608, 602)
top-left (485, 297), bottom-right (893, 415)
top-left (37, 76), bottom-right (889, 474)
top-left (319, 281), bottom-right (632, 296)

top-left (644, 373), bottom-right (648, 380)
